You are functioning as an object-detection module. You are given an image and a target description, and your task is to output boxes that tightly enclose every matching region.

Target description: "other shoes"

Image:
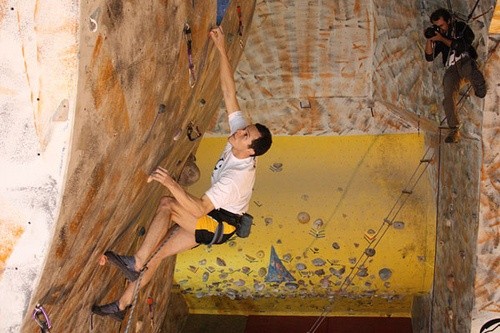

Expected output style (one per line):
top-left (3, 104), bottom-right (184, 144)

top-left (445, 136), bottom-right (459, 144)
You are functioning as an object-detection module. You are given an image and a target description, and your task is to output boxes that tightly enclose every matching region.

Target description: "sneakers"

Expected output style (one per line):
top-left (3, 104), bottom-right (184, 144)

top-left (104, 251), bottom-right (140, 281)
top-left (92, 304), bottom-right (127, 320)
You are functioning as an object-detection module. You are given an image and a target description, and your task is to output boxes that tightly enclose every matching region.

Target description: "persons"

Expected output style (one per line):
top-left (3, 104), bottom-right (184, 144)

top-left (425, 9), bottom-right (486, 143)
top-left (91, 26), bottom-right (272, 320)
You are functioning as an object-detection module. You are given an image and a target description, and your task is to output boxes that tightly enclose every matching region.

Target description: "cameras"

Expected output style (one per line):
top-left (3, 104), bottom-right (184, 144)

top-left (424, 24), bottom-right (441, 39)
